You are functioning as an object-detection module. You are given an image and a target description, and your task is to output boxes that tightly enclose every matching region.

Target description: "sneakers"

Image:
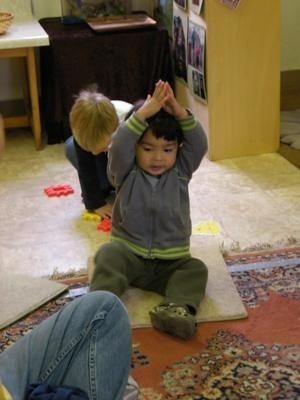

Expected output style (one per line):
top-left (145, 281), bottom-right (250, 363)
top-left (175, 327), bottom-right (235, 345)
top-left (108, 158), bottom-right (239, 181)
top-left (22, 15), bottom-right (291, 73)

top-left (148, 302), bottom-right (199, 340)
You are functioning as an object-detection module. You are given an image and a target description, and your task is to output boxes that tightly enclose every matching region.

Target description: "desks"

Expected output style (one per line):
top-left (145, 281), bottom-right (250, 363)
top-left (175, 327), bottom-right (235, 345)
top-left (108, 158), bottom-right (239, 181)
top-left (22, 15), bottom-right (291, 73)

top-left (0, 15), bottom-right (50, 151)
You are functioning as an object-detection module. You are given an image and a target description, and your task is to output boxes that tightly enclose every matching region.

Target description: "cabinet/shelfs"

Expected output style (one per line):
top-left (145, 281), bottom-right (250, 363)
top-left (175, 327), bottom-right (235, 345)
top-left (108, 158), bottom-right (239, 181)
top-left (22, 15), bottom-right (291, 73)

top-left (39, 11), bottom-right (169, 143)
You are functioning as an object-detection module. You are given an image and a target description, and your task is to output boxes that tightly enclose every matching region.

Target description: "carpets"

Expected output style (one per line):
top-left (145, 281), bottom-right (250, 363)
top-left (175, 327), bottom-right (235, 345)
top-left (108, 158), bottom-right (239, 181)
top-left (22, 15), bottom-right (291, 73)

top-left (0, 240), bottom-right (300, 400)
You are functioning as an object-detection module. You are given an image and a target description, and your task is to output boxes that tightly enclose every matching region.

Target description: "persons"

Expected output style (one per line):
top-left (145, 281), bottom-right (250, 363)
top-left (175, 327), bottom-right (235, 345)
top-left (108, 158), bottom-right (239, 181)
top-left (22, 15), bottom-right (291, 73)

top-left (88, 79), bottom-right (210, 341)
top-left (194, 42), bottom-right (201, 67)
top-left (64, 82), bottom-right (134, 220)
top-left (0, 288), bottom-right (135, 400)
